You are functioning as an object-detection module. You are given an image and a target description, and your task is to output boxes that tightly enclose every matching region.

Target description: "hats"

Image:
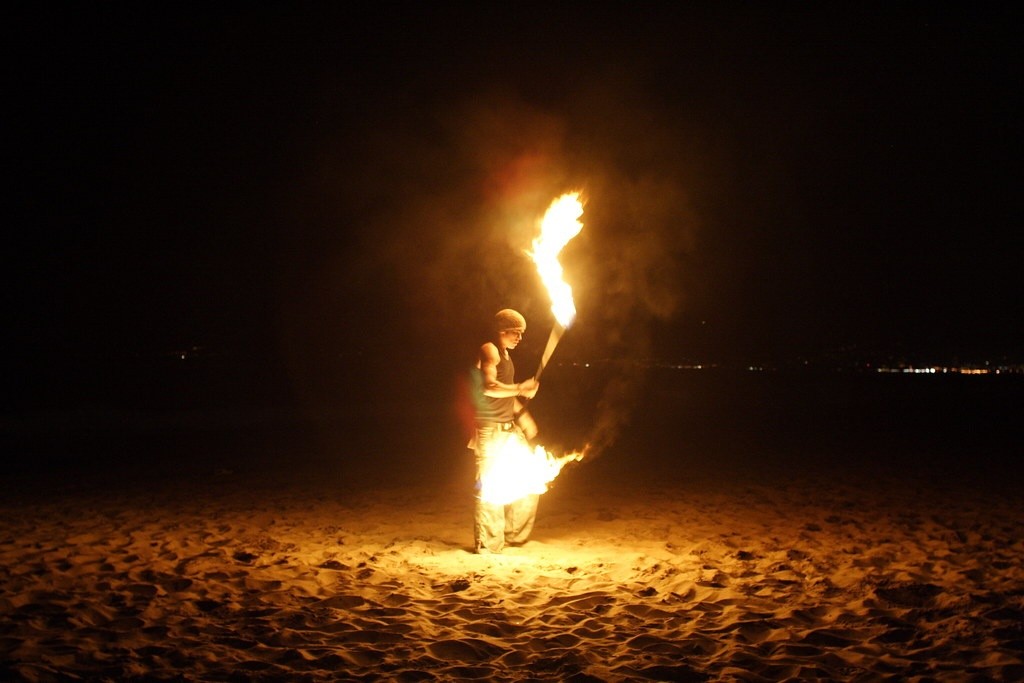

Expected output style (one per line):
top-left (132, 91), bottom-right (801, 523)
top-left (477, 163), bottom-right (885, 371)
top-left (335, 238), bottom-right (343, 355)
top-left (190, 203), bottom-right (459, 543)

top-left (494, 308), bottom-right (526, 332)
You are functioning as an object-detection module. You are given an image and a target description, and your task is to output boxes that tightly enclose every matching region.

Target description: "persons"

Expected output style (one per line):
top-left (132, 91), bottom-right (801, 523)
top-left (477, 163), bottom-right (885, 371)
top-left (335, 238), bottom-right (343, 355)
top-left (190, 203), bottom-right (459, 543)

top-left (466, 310), bottom-right (541, 555)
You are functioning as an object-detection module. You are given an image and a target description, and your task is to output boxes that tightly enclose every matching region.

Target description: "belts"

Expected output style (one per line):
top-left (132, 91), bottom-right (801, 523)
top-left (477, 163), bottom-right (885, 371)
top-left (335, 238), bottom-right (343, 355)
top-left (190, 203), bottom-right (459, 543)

top-left (475, 421), bottom-right (515, 431)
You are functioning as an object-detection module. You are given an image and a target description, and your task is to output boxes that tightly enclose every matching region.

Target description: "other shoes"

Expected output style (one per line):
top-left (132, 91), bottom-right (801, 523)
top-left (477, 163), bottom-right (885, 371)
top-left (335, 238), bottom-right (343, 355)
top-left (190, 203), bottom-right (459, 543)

top-left (474, 542), bottom-right (487, 554)
